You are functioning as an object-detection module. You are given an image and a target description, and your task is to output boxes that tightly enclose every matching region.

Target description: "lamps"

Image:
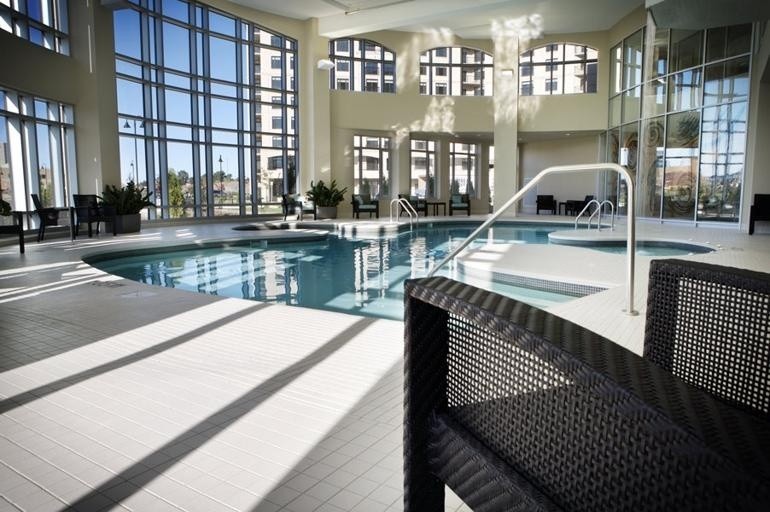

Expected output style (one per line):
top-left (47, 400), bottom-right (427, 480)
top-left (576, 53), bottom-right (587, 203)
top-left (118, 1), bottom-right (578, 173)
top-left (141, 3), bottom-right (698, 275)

top-left (318, 59), bottom-right (334, 71)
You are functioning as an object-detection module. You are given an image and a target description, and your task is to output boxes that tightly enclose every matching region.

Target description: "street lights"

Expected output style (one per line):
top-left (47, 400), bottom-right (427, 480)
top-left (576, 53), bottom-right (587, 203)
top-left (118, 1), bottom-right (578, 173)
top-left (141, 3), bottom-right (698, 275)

top-left (123, 115), bottom-right (146, 190)
top-left (218, 155), bottom-right (223, 207)
top-left (41, 163), bottom-right (48, 186)
top-left (130, 163), bottom-right (135, 182)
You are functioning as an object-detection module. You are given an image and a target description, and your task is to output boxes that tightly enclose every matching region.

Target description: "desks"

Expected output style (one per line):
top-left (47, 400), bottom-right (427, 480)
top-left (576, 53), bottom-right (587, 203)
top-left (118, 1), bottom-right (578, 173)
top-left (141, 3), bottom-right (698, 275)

top-left (427, 201), bottom-right (446, 216)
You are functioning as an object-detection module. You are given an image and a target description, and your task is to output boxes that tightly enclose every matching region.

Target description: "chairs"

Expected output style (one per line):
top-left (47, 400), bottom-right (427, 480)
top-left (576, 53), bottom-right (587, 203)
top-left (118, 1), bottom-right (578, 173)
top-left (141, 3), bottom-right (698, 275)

top-left (350, 194), bottom-right (379, 218)
top-left (282, 193), bottom-right (315, 221)
top-left (31, 194), bottom-right (75, 242)
top-left (566, 195), bottom-right (593, 217)
top-left (449, 194), bottom-right (471, 216)
top-left (748, 193), bottom-right (770, 235)
top-left (0, 211), bottom-right (24, 253)
top-left (73, 195), bottom-right (116, 238)
top-left (403, 257), bottom-right (769, 512)
top-left (536, 195), bottom-right (556, 214)
top-left (399, 194), bottom-right (427, 216)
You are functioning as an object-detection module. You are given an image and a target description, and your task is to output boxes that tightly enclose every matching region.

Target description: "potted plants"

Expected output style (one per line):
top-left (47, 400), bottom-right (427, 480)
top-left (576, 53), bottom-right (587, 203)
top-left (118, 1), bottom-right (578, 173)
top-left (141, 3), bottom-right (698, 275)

top-left (305, 180), bottom-right (348, 219)
top-left (96, 181), bottom-right (157, 233)
